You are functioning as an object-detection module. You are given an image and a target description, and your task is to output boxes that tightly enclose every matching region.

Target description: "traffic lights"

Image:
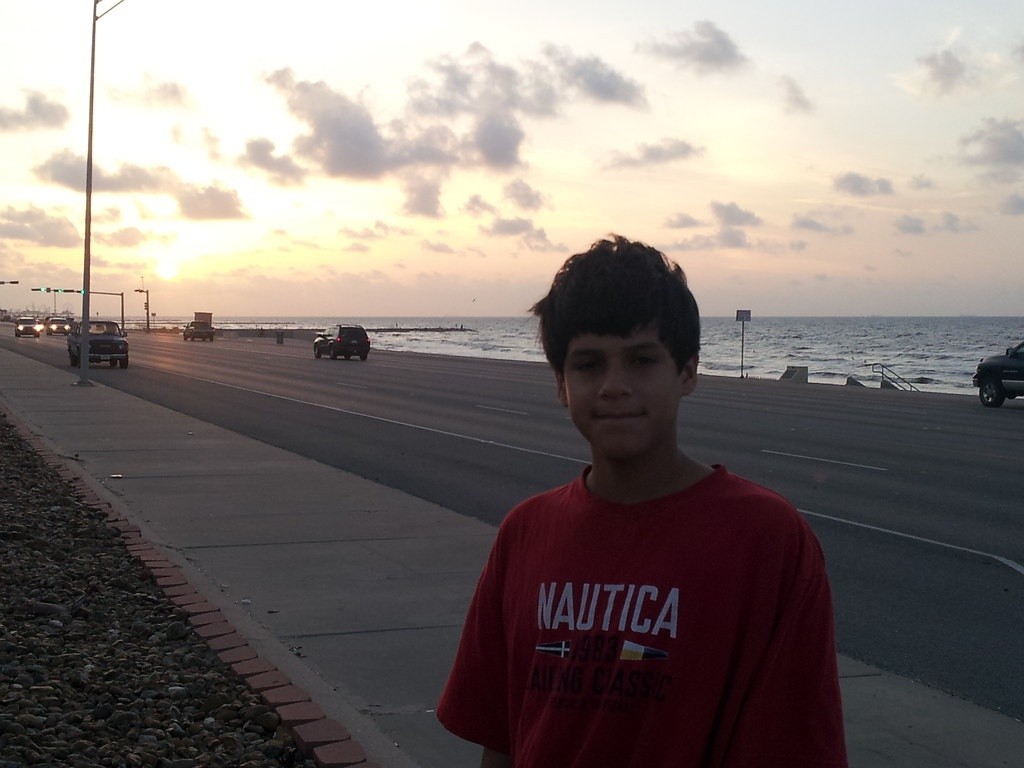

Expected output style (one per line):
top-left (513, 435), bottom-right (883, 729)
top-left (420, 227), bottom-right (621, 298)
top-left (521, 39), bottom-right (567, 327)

top-left (41, 287), bottom-right (45, 292)
top-left (54, 289), bottom-right (62, 293)
top-left (145, 302), bottom-right (147, 310)
top-left (77, 290), bottom-right (85, 294)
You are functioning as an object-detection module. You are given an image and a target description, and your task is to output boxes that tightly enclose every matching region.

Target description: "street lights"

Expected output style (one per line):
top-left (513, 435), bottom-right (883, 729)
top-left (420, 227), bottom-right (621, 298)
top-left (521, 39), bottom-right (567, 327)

top-left (135, 289), bottom-right (149, 330)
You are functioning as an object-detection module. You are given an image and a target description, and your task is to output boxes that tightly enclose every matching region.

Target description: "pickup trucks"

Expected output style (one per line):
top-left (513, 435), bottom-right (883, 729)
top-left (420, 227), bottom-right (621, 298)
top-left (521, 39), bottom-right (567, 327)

top-left (183, 320), bottom-right (215, 341)
top-left (68, 320), bottom-right (128, 370)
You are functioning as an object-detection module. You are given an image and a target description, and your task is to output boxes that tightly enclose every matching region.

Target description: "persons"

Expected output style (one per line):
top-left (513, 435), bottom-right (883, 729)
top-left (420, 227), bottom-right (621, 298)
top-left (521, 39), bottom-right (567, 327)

top-left (437, 236), bottom-right (848, 768)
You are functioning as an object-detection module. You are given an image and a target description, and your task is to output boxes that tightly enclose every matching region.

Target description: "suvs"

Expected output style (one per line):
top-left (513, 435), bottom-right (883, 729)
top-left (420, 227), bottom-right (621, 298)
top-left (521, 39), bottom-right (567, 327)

top-left (15, 317), bottom-right (39, 338)
top-left (47, 318), bottom-right (70, 336)
top-left (313, 324), bottom-right (370, 360)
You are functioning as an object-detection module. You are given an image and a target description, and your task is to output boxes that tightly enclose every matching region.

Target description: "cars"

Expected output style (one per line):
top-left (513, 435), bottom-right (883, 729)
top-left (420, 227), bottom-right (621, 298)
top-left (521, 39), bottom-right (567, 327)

top-left (970, 340), bottom-right (1023, 406)
top-left (36, 320), bottom-right (44, 332)
